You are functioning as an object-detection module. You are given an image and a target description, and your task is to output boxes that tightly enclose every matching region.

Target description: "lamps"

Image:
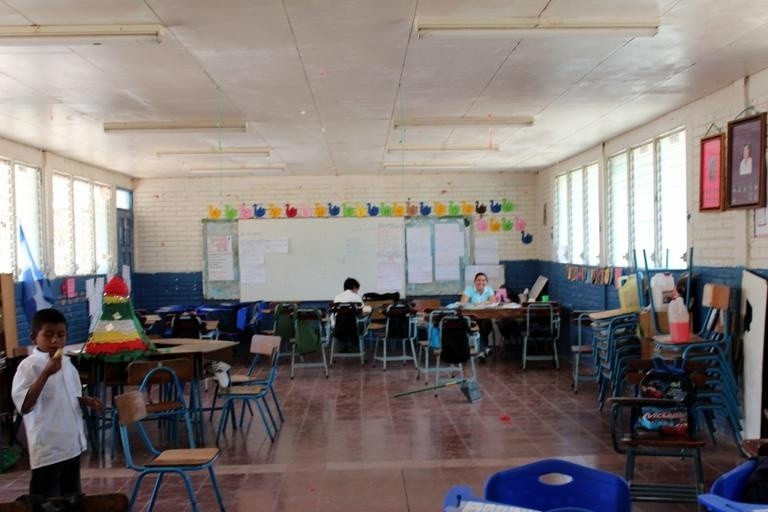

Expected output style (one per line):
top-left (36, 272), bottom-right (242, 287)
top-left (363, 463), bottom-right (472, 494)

top-left (155, 147), bottom-right (272, 158)
top-left (0, 23), bottom-right (165, 45)
top-left (392, 116), bottom-right (535, 129)
top-left (413, 16), bottom-right (660, 41)
top-left (188, 162), bottom-right (287, 172)
top-left (386, 144), bottom-right (500, 156)
top-left (102, 120), bottom-right (249, 134)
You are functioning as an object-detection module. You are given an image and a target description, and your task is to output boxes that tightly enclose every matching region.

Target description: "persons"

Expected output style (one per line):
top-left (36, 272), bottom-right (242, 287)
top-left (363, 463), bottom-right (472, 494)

top-left (11, 308), bottom-right (101, 497)
top-left (492, 283), bottom-right (521, 345)
top-left (738, 144), bottom-right (753, 176)
top-left (328, 277), bottom-right (365, 329)
top-left (459, 272), bottom-right (496, 359)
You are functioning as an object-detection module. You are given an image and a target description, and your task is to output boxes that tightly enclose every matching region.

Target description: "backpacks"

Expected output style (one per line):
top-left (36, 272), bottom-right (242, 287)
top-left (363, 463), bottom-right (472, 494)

top-left (293, 307), bottom-right (322, 356)
top-left (252, 300), bottom-right (273, 331)
top-left (331, 304), bottom-right (356, 342)
top-left (387, 304), bottom-right (411, 340)
top-left (274, 304), bottom-right (296, 338)
top-left (439, 319), bottom-right (471, 364)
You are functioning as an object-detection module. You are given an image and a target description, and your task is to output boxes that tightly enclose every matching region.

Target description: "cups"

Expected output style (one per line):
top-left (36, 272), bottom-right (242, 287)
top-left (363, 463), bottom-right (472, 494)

top-left (541, 295), bottom-right (549, 306)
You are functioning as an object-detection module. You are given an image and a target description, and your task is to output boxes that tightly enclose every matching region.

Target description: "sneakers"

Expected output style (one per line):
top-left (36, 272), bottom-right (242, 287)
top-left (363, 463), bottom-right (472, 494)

top-left (479, 348), bottom-right (493, 359)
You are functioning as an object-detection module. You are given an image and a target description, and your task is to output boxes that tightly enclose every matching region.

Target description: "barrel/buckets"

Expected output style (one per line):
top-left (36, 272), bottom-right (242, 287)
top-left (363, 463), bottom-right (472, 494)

top-left (650, 272), bottom-right (675, 312)
top-left (617, 274), bottom-right (642, 311)
top-left (667, 297), bottom-right (689, 343)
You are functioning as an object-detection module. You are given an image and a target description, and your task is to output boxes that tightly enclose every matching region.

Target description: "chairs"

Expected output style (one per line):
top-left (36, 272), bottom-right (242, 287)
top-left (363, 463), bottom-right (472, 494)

top-left (441, 458), bottom-right (632, 512)
top-left (695, 454), bottom-right (768, 512)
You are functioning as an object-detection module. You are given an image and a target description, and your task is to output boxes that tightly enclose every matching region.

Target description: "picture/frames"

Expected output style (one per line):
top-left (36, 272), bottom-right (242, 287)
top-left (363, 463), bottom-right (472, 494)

top-left (725, 112), bottom-right (767, 210)
top-left (699, 131), bottom-right (725, 211)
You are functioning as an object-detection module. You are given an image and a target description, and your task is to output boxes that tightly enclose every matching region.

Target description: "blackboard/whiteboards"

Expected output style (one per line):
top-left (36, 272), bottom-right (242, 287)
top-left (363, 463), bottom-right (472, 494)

top-left (201, 215), bottom-right (474, 303)
